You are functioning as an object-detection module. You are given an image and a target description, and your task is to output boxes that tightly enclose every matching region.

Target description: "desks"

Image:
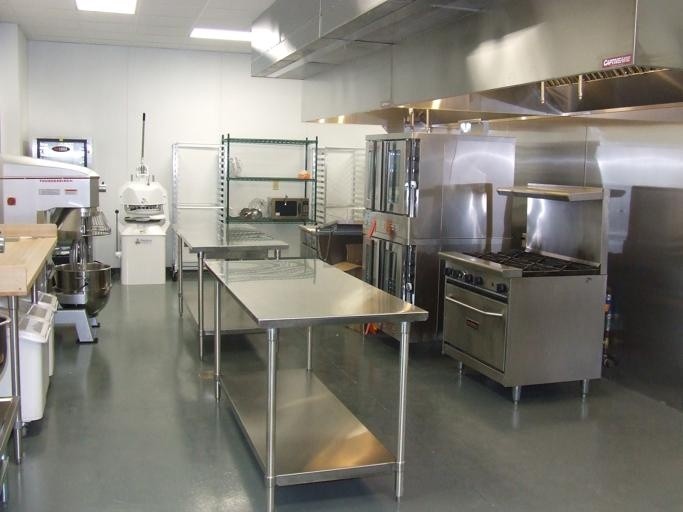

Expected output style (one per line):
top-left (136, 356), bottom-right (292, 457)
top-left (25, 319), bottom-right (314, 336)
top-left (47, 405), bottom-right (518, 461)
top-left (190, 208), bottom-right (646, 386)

top-left (0, 221), bottom-right (59, 466)
top-left (298, 224), bottom-right (364, 261)
top-left (168, 223), bottom-right (289, 361)
top-left (203, 257), bottom-right (429, 512)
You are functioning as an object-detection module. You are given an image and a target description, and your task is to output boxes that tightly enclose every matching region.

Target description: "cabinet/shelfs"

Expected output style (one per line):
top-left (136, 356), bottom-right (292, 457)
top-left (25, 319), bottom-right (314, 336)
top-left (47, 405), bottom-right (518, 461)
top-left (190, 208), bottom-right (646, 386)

top-left (169, 133), bottom-right (318, 281)
top-left (309, 146), bottom-right (367, 228)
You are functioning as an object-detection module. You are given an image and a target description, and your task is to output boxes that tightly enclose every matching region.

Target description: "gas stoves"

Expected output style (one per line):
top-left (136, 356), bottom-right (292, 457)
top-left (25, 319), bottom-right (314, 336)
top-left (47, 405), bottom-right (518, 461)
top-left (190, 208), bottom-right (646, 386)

top-left (437, 248), bottom-right (600, 296)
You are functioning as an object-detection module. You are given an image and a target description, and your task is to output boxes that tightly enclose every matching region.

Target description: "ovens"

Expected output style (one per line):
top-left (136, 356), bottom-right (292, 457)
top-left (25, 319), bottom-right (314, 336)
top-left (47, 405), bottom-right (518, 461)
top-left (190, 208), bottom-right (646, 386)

top-left (442, 280), bottom-right (508, 371)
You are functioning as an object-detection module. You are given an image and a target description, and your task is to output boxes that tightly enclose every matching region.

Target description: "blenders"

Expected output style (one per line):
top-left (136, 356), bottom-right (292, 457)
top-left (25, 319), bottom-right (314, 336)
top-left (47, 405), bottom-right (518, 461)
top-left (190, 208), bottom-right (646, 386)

top-left (0, 153), bottom-right (114, 323)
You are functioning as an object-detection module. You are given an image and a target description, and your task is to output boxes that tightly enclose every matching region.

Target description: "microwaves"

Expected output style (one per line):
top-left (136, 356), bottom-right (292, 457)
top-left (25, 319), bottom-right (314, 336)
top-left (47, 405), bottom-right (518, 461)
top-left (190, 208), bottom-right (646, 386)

top-left (267, 196), bottom-right (309, 219)
